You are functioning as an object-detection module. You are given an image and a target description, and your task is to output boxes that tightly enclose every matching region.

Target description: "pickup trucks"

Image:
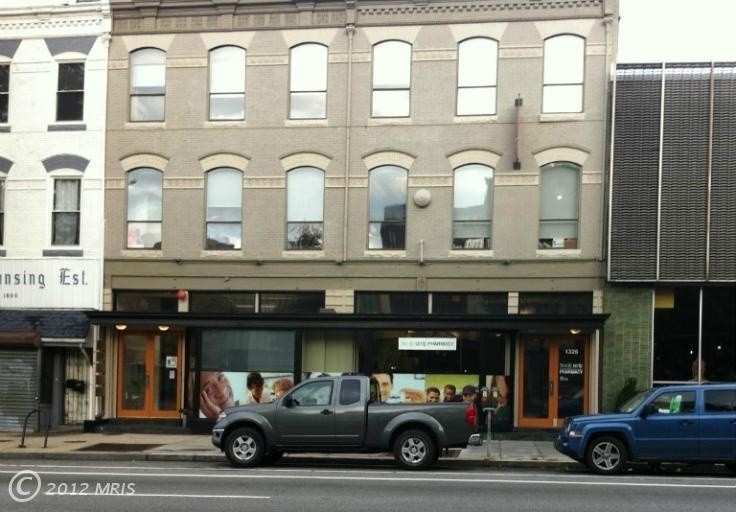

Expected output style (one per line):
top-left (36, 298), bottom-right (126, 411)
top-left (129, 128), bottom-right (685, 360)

top-left (209, 374), bottom-right (482, 471)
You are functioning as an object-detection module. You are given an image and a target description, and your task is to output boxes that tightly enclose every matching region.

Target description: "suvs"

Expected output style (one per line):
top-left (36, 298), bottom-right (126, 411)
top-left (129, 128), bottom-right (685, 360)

top-left (543, 386), bottom-right (583, 417)
top-left (551, 382), bottom-right (735, 474)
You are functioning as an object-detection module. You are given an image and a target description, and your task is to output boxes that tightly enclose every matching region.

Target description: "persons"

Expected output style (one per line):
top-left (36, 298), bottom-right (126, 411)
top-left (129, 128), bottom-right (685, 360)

top-left (463, 386), bottom-right (483, 408)
top-left (273, 379), bottom-right (293, 399)
top-left (425, 387), bottom-right (442, 403)
top-left (443, 384), bottom-right (463, 403)
top-left (200, 372), bottom-right (234, 421)
top-left (373, 373), bottom-right (393, 403)
top-left (245, 373), bottom-right (271, 405)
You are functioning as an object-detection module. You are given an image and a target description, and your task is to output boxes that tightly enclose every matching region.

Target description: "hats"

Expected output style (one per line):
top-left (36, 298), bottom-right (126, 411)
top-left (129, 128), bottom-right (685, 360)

top-left (462, 385), bottom-right (475, 395)
